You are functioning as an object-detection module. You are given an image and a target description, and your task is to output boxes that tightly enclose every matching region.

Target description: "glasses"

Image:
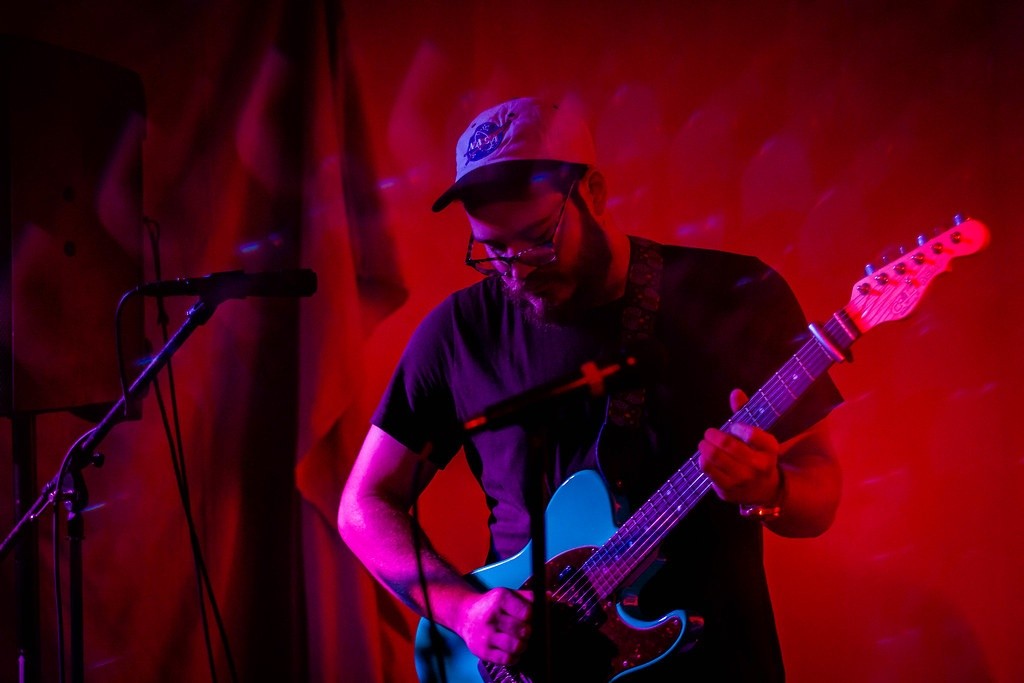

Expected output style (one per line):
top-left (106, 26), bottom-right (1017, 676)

top-left (465, 182), bottom-right (574, 276)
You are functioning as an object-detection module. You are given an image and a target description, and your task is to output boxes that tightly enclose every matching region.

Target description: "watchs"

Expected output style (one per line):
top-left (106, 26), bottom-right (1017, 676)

top-left (740, 463), bottom-right (790, 521)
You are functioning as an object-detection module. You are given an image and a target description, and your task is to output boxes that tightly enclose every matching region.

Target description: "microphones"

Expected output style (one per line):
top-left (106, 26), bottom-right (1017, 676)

top-left (136, 267), bottom-right (319, 298)
top-left (460, 357), bottom-right (636, 437)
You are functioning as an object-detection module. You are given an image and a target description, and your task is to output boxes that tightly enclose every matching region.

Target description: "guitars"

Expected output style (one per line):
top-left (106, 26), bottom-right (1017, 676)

top-left (413, 215), bottom-right (990, 683)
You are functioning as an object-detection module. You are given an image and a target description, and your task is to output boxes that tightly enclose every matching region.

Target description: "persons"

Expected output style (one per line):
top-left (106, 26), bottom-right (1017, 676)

top-left (336, 95), bottom-right (840, 683)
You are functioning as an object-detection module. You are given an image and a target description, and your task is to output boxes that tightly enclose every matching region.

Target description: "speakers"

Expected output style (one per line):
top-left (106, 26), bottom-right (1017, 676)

top-left (0, 34), bottom-right (147, 417)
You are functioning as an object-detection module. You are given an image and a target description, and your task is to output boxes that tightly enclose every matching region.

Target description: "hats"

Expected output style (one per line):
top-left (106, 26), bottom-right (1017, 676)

top-left (432, 96), bottom-right (590, 211)
